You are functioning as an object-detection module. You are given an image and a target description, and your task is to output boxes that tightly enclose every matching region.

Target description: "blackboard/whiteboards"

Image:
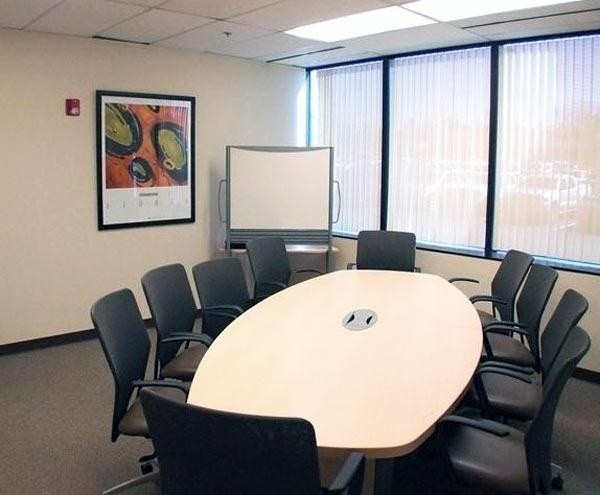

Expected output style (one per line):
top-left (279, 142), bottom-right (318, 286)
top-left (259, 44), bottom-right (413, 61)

top-left (224, 146), bottom-right (333, 245)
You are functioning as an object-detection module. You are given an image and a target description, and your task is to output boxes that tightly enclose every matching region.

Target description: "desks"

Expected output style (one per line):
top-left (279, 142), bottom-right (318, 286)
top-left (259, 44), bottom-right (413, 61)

top-left (184, 268), bottom-right (483, 494)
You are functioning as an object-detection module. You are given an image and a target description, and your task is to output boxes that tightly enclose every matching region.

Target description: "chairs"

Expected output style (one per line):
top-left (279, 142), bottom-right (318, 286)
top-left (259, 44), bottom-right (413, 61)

top-left (136, 387), bottom-right (368, 494)
top-left (394, 247), bottom-right (591, 495)
top-left (90, 289), bottom-right (190, 477)
top-left (141, 235), bottom-right (327, 381)
top-left (346, 230), bottom-right (422, 273)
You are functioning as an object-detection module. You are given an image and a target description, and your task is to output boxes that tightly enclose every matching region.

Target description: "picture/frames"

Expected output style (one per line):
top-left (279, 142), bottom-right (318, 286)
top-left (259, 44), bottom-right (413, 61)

top-left (96, 90), bottom-right (196, 229)
